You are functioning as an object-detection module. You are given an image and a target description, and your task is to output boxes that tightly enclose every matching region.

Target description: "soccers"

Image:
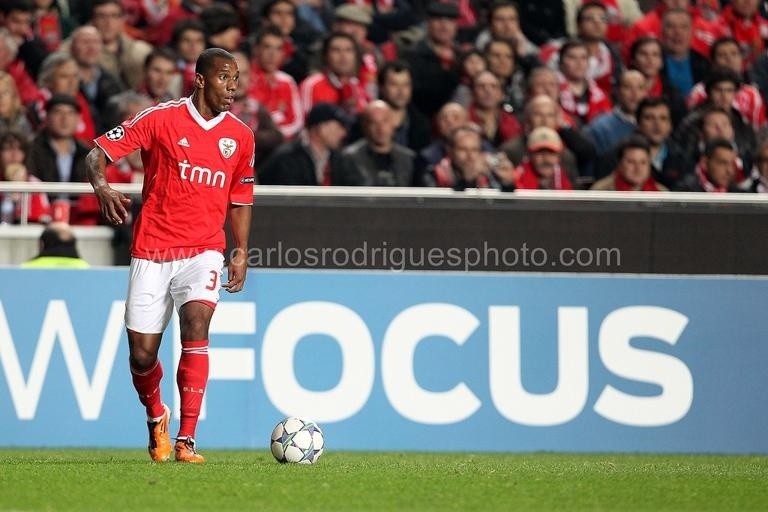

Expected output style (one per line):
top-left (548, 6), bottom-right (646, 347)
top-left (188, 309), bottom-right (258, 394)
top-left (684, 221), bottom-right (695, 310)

top-left (271, 417), bottom-right (324, 464)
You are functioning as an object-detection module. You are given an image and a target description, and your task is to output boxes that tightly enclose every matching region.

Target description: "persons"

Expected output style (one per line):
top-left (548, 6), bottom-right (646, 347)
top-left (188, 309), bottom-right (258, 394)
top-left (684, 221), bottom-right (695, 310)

top-left (23, 220), bottom-right (91, 270)
top-left (1, 1), bottom-right (767, 231)
top-left (82, 47), bottom-right (260, 466)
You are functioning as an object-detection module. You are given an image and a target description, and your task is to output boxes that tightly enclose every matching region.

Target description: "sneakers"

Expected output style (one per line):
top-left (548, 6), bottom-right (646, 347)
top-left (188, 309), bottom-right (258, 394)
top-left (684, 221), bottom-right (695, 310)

top-left (145, 403), bottom-right (172, 462)
top-left (174, 437), bottom-right (203, 464)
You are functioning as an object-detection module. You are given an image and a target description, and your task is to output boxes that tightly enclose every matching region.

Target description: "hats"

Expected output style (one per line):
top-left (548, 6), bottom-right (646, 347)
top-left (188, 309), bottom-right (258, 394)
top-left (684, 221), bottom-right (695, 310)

top-left (42, 94), bottom-right (82, 114)
top-left (527, 125), bottom-right (565, 152)
top-left (335, 5), bottom-right (374, 25)
top-left (429, 3), bottom-right (461, 17)
